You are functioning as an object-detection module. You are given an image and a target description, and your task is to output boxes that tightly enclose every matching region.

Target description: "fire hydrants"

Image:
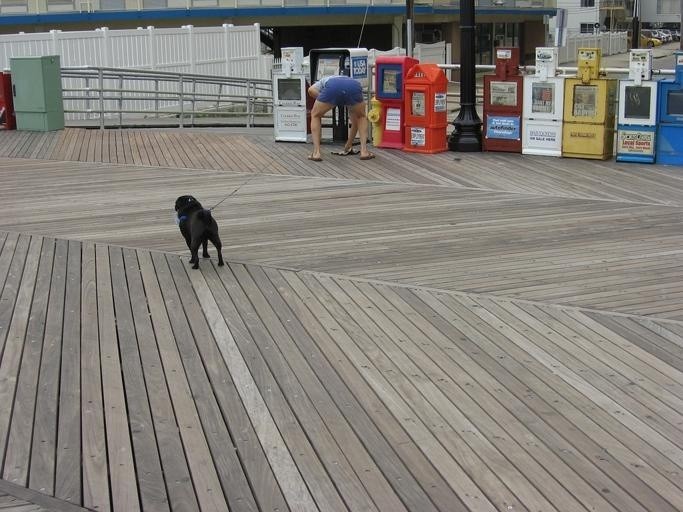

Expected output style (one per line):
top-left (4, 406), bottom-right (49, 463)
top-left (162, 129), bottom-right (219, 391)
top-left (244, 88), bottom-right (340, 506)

top-left (368, 96), bottom-right (383, 146)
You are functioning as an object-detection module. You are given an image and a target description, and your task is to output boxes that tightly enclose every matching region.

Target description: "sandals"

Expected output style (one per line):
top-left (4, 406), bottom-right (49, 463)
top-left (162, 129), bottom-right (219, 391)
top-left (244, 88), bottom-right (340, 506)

top-left (360, 151), bottom-right (374, 160)
top-left (307, 153), bottom-right (322, 160)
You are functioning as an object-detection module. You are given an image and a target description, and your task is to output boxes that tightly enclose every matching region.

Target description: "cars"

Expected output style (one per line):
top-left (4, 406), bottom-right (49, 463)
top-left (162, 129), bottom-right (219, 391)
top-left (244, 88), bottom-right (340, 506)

top-left (627, 29), bottom-right (680, 48)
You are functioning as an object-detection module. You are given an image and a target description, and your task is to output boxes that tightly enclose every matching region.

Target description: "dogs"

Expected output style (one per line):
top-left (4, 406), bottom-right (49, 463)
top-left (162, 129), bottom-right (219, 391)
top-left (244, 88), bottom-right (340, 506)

top-left (174, 194), bottom-right (224, 269)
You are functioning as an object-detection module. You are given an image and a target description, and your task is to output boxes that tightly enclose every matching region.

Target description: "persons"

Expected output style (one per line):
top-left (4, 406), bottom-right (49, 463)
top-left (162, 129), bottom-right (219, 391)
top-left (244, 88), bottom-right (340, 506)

top-left (308, 75), bottom-right (376, 161)
